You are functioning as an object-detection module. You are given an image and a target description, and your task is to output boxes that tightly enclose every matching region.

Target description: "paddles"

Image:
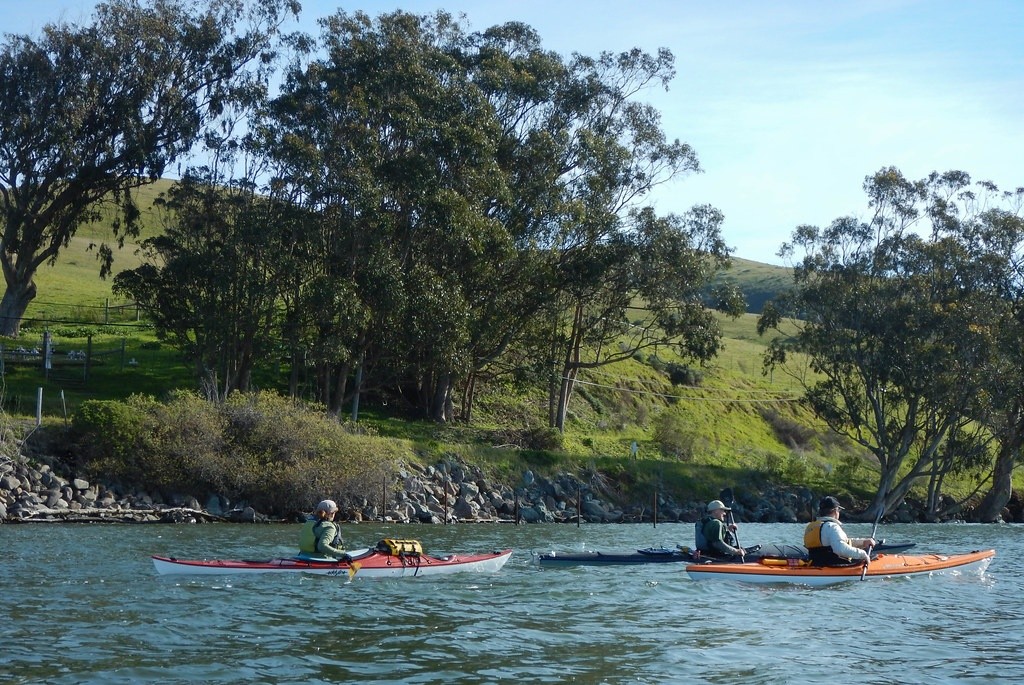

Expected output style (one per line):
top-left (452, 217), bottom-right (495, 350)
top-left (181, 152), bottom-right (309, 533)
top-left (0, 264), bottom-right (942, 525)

top-left (341, 539), bottom-right (364, 578)
top-left (720, 486), bottom-right (747, 564)
top-left (859, 501), bottom-right (888, 581)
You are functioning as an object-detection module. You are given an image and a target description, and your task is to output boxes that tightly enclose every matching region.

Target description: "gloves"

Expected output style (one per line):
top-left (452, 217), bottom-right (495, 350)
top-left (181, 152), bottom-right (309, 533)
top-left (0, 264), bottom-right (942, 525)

top-left (342, 552), bottom-right (353, 563)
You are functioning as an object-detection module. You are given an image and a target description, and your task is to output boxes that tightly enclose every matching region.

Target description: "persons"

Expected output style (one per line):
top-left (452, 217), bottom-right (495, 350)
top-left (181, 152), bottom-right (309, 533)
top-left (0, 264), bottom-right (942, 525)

top-left (302, 499), bottom-right (354, 565)
top-left (803, 495), bottom-right (875, 568)
top-left (699, 499), bottom-right (746, 562)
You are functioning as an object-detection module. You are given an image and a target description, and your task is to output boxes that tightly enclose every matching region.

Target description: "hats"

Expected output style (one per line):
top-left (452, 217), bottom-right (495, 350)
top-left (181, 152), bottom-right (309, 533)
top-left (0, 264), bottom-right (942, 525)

top-left (317, 500), bottom-right (339, 512)
top-left (707, 500), bottom-right (731, 512)
top-left (819, 496), bottom-right (846, 511)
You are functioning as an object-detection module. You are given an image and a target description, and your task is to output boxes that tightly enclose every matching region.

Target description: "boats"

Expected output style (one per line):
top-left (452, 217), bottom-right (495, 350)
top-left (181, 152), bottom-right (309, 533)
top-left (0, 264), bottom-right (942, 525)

top-left (538, 542), bottom-right (918, 567)
top-left (151, 549), bottom-right (513, 577)
top-left (686, 549), bottom-right (995, 586)
